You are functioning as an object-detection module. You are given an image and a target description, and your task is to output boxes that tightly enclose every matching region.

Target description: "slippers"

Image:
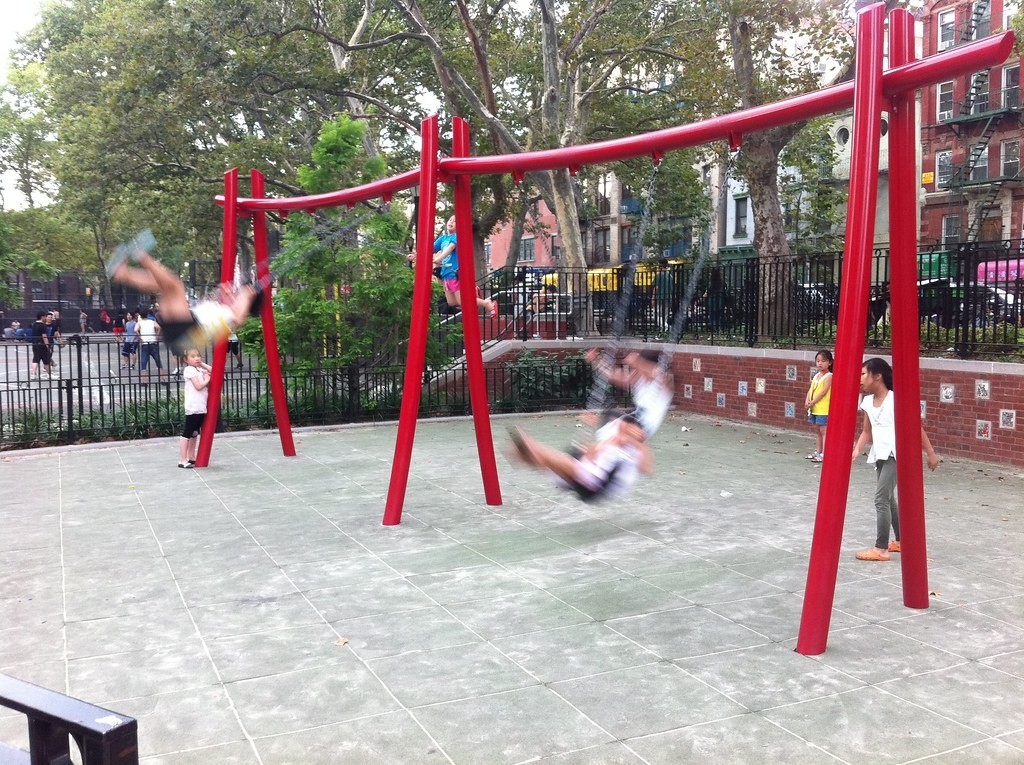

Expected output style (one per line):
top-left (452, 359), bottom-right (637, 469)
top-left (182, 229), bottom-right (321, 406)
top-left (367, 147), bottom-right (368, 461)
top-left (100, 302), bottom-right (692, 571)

top-left (475, 285), bottom-right (483, 301)
top-left (855, 549), bottom-right (891, 561)
top-left (888, 541), bottom-right (901, 552)
top-left (489, 301), bottom-right (498, 320)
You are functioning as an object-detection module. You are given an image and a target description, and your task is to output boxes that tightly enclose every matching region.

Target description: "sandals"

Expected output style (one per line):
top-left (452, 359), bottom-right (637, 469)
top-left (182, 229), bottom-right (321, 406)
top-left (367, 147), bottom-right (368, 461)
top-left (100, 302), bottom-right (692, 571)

top-left (811, 453), bottom-right (824, 462)
top-left (804, 451), bottom-right (818, 459)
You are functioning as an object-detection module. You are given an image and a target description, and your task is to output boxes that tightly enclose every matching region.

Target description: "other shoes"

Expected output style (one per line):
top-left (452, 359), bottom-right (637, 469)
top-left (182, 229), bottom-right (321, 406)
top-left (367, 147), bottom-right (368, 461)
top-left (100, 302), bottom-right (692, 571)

top-left (130, 364), bottom-right (135, 370)
top-left (178, 461), bottom-right (193, 469)
top-left (121, 364), bottom-right (128, 368)
top-left (47, 374), bottom-right (59, 380)
top-left (31, 374), bottom-right (37, 380)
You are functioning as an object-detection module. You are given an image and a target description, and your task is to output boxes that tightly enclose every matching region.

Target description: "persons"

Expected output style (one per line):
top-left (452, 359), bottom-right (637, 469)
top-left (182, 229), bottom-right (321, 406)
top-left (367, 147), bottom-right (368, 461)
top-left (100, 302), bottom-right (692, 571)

top-left (226, 333), bottom-right (244, 368)
top-left (113, 311), bottom-right (126, 338)
top-left (134, 311), bottom-right (166, 386)
top-left (509, 346), bottom-right (674, 498)
top-left (107, 229), bottom-right (265, 355)
top-left (511, 275), bottom-right (529, 317)
top-left (652, 258), bottom-right (673, 331)
top-left (852, 357), bottom-right (938, 561)
top-left (407, 214), bottom-right (498, 318)
top-left (526, 285), bottom-right (557, 315)
top-left (171, 350), bottom-right (184, 374)
top-left (696, 269), bottom-right (723, 330)
top-left (178, 346), bottom-right (212, 468)
top-left (120, 311), bottom-right (139, 370)
top-left (47, 308), bottom-right (59, 322)
top-left (98, 309), bottom-right (111, 333)
top-left (134, 303), bottom-right (159, 320)
top-left (804, 350), bottom-right (834, 462)
top-left (79, 309), bottom-right (95, 335)
top-left (11, 319), bottom-right (33, 341)
top-left (30, 311), bottom-right (62, 380)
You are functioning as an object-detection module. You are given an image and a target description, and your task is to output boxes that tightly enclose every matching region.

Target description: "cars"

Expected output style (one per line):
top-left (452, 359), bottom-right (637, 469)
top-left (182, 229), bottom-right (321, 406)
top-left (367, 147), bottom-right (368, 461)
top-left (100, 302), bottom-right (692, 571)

top-left (794, 276), bottom-right (1022, 331)
